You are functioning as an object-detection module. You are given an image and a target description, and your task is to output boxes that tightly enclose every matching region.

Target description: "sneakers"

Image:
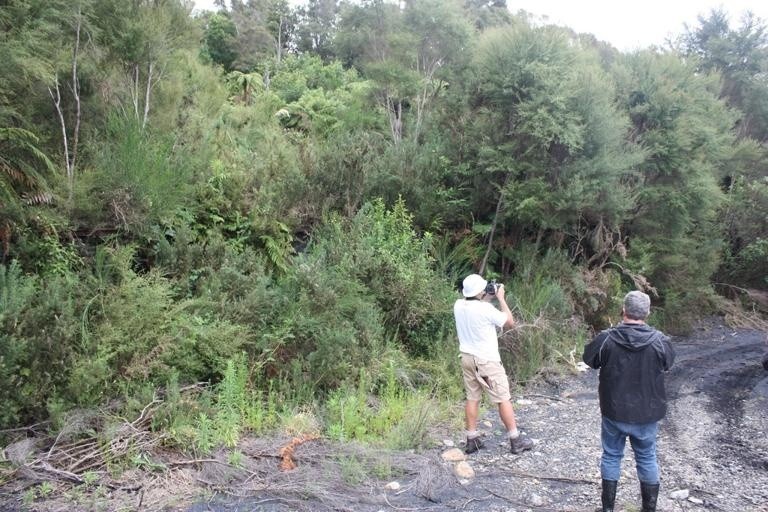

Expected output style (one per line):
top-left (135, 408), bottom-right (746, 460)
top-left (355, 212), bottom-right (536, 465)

top-left (466, 438), bottom-right (486, 455)
top-left (510, 434), bottom-right (534, 454)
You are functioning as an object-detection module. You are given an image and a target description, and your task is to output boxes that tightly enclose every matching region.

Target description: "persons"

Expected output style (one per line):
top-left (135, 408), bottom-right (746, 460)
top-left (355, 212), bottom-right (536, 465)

top-left (453, 272), bottom-right (534, 455)
top-left (582, 289), bottom-right (677, 511)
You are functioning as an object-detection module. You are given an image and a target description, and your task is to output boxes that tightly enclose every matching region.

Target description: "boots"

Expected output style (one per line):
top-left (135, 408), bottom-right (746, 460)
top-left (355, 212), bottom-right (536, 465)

top-left (596, 480), bottom-right (616, 511)
top-left (634, 482), bottom-right (659, 511)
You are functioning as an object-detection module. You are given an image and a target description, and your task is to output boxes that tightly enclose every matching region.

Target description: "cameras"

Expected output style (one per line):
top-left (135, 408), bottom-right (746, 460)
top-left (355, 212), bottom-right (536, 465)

top-left (484, 279), bottom-right (500, 295)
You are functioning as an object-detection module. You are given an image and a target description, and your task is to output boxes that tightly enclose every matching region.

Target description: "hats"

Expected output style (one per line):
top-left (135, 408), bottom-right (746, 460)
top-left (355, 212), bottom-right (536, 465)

top-left (461, 274), bottom-right (487, 298)
top-left (624, 290), bottom-right (651, 320)
